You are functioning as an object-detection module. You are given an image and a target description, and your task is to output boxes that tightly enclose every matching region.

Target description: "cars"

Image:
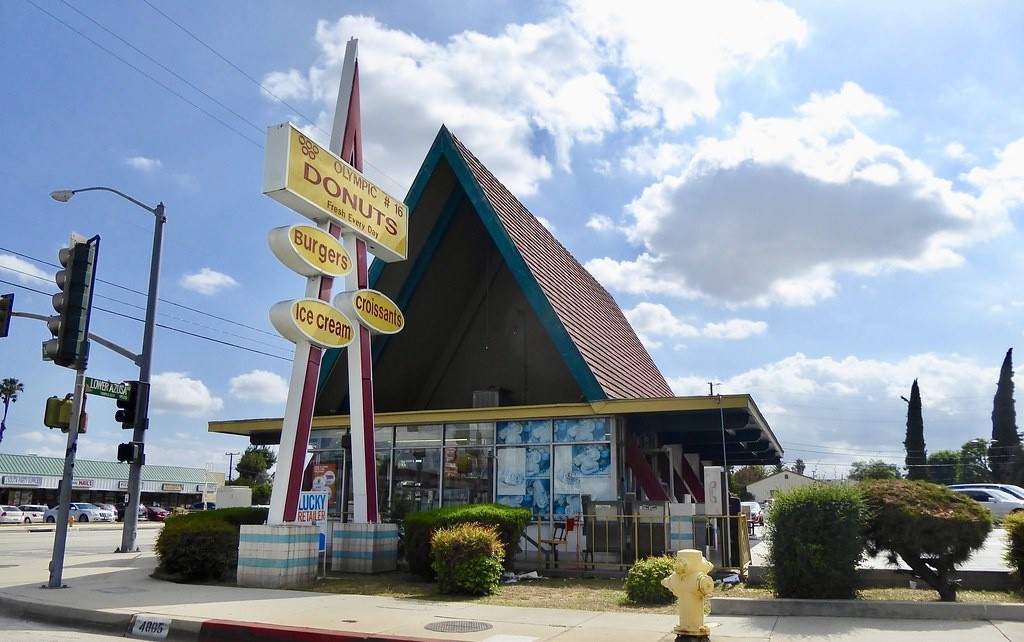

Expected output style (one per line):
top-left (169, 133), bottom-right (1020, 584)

top-left (945, 483), bottom-right (1024, 522)
top-left (740, 501), bottom-right (765, 525)
top-left (43, 502), bottom-right (215, 523)
top-left (0, 505), bottom-right (24, 524)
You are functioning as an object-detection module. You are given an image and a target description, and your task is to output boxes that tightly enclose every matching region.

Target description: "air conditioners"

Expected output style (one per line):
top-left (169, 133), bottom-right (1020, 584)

top-left (472, 390), bottom-right (509, 408)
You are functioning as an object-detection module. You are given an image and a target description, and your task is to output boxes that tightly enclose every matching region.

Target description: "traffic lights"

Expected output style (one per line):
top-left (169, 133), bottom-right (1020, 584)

top-left (43, 395), bottom-right (74, 431)
top-left (707, 382), bottom-right (723, 396)
top-left (118, 442), bottom-right (139, 464)
top-left (1, 295), bottom-right (12, 336)
top-left (46, 243), bottom-right (96, 370)
top-left (115, 382), bottom-right (146, 430)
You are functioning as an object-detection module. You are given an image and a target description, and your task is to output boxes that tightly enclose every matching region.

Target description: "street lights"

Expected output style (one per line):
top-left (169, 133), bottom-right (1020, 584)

top-left (225, 451), bottom-right (239, 485)
top-left (50, 187), bottom-right (167, 554)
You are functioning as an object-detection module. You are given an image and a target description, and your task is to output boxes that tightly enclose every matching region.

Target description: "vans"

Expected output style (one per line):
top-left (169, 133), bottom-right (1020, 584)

top-left (17, 505), bottom-right (49, 523)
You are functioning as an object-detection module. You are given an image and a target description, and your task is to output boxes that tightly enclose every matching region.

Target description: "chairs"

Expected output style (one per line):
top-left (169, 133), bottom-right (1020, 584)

top-left (541, 522), bottom-right (568, 547)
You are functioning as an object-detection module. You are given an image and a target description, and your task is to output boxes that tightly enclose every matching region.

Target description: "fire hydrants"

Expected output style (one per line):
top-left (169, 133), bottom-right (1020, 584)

top-left (69, 516), bottom-right (76, 527)
top-left (661, 549), bottom-right (714, 638)
